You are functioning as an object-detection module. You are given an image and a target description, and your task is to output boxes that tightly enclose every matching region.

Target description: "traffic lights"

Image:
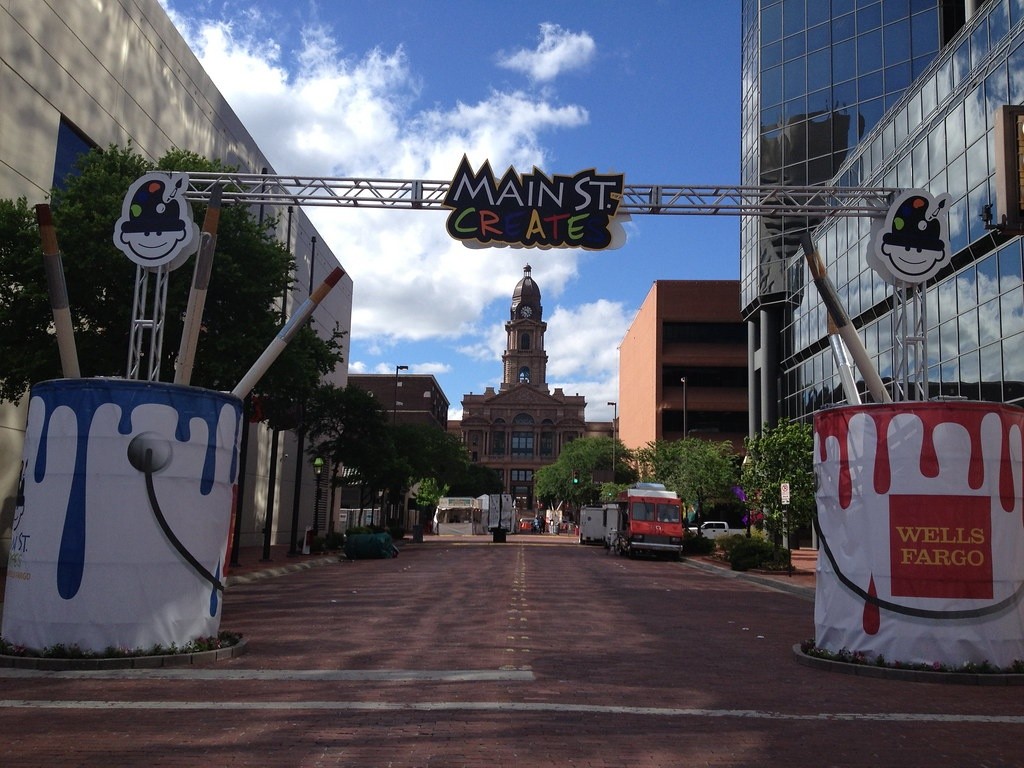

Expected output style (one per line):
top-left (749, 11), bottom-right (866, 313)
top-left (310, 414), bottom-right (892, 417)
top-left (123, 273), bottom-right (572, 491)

top-left (573, 470), bottom-right (579, 484)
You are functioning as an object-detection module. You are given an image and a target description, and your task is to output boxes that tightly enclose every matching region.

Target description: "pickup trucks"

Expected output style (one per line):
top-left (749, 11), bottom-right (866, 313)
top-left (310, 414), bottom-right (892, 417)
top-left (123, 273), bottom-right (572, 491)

top-left (689, 521), bottom-right (746, 539)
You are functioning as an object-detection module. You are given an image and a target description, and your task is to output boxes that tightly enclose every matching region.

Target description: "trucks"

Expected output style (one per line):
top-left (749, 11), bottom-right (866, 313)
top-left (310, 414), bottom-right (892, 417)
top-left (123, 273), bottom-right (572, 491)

top-left (602, 483), bottom-right (689, 561)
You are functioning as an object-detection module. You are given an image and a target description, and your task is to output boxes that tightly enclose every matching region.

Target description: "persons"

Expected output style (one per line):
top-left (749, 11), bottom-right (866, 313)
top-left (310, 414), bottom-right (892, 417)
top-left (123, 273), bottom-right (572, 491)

top-left (534, 515), bottom-right (546, 533)
top-left (465, 513), bottom-right (470, 520)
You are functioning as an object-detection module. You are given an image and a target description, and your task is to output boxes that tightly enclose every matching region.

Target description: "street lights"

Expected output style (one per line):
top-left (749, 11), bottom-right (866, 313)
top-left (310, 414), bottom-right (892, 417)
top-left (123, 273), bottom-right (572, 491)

top-left (313, 457), bottom-right (324, 536)
top-left (608, 402), bottom-right (616, 470)
top-left (742, 452), bottom-right (752, 538)
top-left (393, 366), bottom-right (409, 426)
top-left (681, 377), bottom-right (687, 438)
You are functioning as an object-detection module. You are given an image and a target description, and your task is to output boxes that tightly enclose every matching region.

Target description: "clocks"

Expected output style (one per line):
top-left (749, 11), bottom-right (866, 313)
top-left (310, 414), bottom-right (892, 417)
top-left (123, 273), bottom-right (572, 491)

top-left (520, 305), bottom-right (533, 319)
